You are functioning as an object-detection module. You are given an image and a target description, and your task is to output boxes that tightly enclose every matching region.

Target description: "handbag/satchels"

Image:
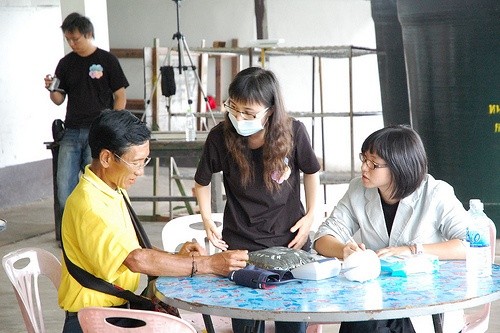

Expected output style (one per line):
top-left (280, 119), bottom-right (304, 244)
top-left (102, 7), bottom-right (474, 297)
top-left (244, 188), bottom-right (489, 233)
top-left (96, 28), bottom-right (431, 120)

top-left (130, 275), bottom-right (181, 319)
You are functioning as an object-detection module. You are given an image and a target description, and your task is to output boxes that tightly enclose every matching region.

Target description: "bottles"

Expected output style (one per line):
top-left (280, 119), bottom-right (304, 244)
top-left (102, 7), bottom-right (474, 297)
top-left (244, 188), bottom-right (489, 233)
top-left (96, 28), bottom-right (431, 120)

top-left (464, 199), bottom-right (493, 279)
top-left (184, 109), bottom-right (196, 142)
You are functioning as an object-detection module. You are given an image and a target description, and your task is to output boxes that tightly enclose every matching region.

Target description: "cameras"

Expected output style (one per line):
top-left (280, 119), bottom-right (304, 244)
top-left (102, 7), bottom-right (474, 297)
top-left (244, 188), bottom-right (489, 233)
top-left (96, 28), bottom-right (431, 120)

top-left (48, 77), bottom-right (66, 94)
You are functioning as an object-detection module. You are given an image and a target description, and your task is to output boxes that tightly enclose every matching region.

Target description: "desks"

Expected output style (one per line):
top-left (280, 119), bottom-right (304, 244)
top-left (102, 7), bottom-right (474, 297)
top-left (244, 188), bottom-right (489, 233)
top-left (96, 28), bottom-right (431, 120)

top-left (153, 251), bottom-right (500, 333)
top-left (43, 129), bottom-right (227, 241)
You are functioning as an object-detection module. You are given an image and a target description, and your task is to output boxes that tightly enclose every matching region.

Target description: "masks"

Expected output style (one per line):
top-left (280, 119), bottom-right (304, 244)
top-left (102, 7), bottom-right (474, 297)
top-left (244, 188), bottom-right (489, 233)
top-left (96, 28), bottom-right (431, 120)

top-left (228, 107), bottom-right (270, 136)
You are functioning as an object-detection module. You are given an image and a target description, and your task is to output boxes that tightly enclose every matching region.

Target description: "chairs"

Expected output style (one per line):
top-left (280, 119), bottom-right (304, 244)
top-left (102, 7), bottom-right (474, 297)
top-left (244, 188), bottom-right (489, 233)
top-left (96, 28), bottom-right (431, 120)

top-left (162, 212), bottom-right (226, 256)
top-left (77, 307), bottom-right (198, 333)
top-left (1, 247), bottom-right (66, 333)
top-left (460, 218), bottom-right (496, 333)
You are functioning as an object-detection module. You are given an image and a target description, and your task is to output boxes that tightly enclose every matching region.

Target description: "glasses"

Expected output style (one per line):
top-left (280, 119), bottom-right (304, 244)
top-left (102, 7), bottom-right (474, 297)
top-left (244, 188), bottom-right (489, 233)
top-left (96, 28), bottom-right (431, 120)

top-left (223, 98), bottom-right (270, 120)
top-left (112, 152), bottom-right (151, 171)
top-left (65, 34), bottom-right (85, 43)
top-left (359, 152), bottom-right (390, 170)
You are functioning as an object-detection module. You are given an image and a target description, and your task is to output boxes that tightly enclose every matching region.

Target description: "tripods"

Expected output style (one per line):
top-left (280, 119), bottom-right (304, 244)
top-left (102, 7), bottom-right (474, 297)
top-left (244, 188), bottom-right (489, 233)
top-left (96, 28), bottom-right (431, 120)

top-left (141, 0), bottom-right (217, 131)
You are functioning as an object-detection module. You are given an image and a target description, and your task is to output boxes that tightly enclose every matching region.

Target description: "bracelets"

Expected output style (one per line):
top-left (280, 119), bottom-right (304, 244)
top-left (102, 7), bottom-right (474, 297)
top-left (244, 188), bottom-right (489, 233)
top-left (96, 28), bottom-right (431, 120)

top-left (191, 255), bottom-right (194, 277)
top-left (408, 243), bottom-right (425, 255)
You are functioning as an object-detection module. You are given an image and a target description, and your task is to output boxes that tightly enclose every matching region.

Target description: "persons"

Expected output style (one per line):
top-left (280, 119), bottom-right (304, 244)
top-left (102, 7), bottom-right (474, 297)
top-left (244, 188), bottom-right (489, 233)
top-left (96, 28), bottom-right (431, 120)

top-left (58, 109), bottom-right (250, 333)
top-left (44, 13), bottom-right (130, 249)
top-left (194, 68), bottom-right (321, 333)
top-left (311, 125), bottom-right (468, 333)
